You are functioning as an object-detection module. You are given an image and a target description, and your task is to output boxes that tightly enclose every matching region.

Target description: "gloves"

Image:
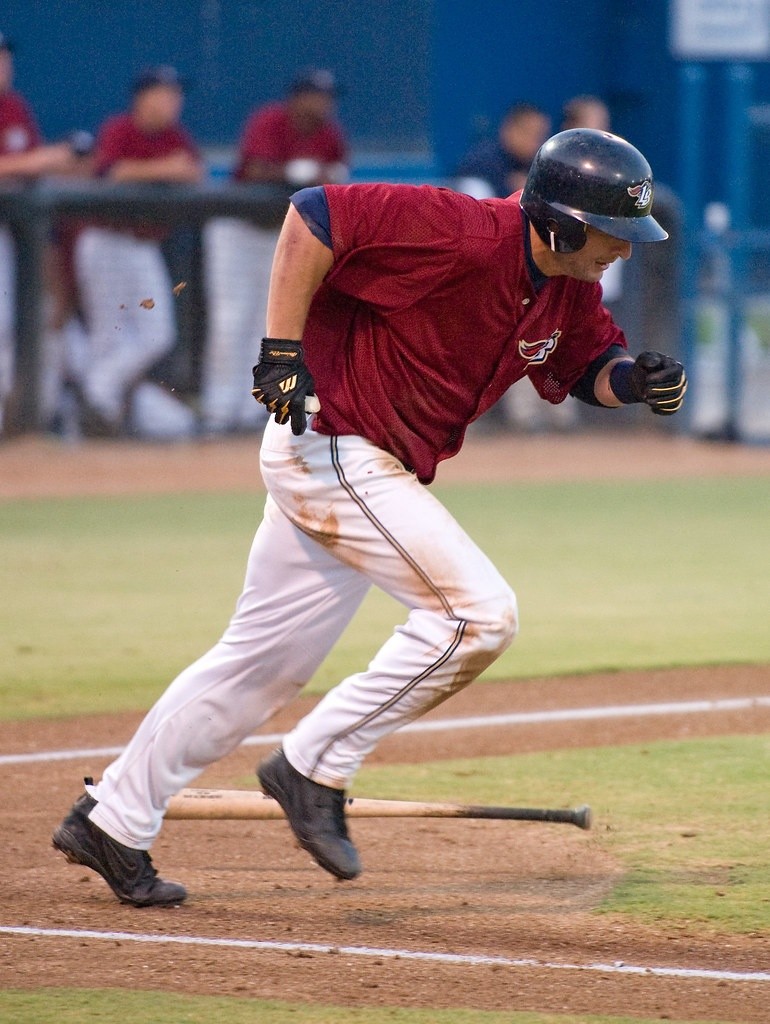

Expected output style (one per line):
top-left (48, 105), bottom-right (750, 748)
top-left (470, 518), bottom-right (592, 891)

top-left (610, 350), bottom-right (689, 416)
top-left (286, 154), bottom-right (321, 190)
top-left (254, 338), bottom-right (323, 439)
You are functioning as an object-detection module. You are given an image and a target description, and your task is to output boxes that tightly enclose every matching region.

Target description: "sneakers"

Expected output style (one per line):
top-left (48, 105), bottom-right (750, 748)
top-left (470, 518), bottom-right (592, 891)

top-left (48, 774), bottom-right (187, 906)
top-left (257, 747), bottom-right (360, 882)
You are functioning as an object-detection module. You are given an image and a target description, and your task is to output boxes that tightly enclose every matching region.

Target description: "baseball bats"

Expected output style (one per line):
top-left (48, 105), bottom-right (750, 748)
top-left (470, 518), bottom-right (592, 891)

top-left (162, 787), bottom-right (592, 830)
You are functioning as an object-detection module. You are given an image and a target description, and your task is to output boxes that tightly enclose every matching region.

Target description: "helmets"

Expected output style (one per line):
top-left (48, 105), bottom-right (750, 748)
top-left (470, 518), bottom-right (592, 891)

top-left (125, 64), bottom-right (195, 96)
top-left (517, 127), bottom-right (670, 252)
top-left (284, 69), bottom-right (350, 105)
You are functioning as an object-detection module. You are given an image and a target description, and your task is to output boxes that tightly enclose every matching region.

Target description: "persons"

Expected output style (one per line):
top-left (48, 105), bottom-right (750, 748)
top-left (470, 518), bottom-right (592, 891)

top-left (49, 127), bottom-right (689, 906)
top-left (0, 42), bottom-right (632, 441)
